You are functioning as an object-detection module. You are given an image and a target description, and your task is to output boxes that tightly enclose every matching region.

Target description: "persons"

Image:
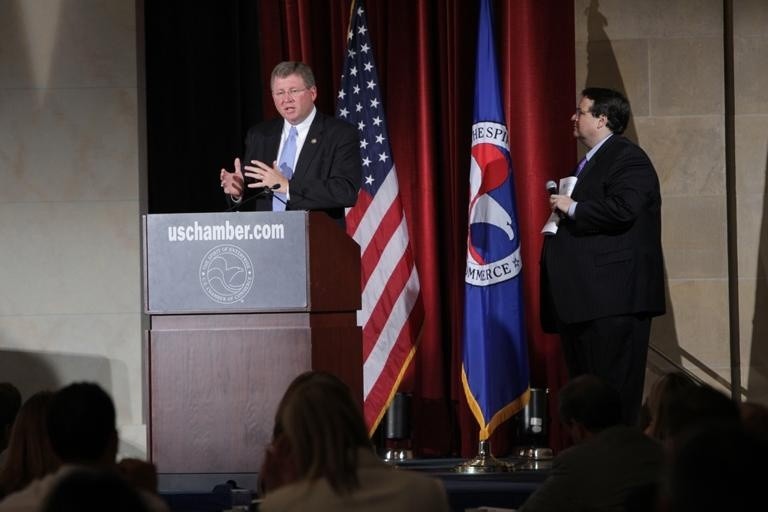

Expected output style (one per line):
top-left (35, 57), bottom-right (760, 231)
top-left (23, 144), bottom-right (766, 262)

top-left (250, 367), bottom-right (449, 511)
top-left (517, 367), bottom-right (768, 512)
top-left (0, 379), bottom-right (172, 511)
top-left (534, 85), bottom-right (669, 430)
top-left (215, 56), bottom-right (361, 234)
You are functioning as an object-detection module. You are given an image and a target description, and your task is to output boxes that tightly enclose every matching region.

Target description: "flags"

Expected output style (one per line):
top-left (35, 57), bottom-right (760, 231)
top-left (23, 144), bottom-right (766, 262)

top-left (459, 0), bottom-right (531, 447)
top-left (327, 1), bottom-right (425, 444)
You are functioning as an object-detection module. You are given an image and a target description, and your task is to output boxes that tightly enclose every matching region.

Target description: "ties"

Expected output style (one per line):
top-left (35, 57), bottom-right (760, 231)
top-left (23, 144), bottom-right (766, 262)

top-left (271, 127), bottom-right (299, 212)
top-left (573, 156), bottom-right (587, 180)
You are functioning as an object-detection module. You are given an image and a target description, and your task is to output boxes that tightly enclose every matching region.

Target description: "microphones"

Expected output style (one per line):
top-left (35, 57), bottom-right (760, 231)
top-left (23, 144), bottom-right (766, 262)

top-left (546, 180), bottom-right (566, 220)
top-left (223, 183), bottom-right (281, 212)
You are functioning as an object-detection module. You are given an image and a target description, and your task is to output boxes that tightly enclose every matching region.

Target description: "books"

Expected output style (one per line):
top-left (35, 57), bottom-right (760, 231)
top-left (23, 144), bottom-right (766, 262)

top-left (539, 175), bottom-right (579, 237)
